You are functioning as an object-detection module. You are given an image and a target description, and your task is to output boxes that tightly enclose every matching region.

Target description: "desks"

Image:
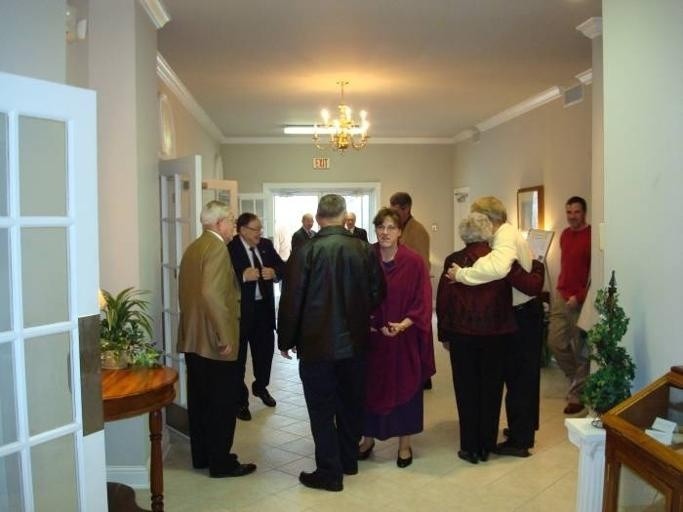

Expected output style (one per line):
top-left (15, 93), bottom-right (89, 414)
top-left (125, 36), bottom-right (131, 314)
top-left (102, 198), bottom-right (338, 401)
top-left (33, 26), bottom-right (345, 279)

top-left (102, 367), bottom-right (179, 512)
top-left (564, 417), bottom-right (666, 511)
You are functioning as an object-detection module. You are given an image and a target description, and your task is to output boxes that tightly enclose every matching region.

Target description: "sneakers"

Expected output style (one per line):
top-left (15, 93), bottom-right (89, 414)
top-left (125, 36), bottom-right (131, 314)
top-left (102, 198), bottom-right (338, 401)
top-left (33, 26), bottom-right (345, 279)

top-left (396, 446), bottom-right (412, 468)
top-left (358, 440), bottom-right (375, 461)
top-left (342, 463), bottom-right (359, 475)
top-left (458, 450), bottom-right (478, 463)
top-left (228, 452), bottom-right (238, 462)
top-left (493, 443), bottom-right (531, 457)
top-left (236, 405), bottom-right (251, 421)
top-left (209, 463), bottom-right (257, 478)
top-left (253, 386), bottom-right (277, 407)
top-left (504, 426), bottom-right (512, 437)
top-left (564, 401), bottom-right (584, 415)
top-left (299, 471), bottom-right (343, 492)
top-left (480, 447), bottom-right (490, 462)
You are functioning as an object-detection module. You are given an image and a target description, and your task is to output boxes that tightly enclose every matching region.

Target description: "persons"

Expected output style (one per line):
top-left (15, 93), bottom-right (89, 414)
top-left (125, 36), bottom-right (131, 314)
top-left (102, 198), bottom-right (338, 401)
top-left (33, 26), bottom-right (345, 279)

top-left (358, 207), bottom-right (433, 469)
top-left (291, 213), bottom-right (318, 250)
top-left (175, 199), bottom-right (258, 479)
top-left (546, 196), bottom-right (591, 415)
top-left (345, 212), bottom-right (368, 243)
top-left (435, 213), bottom-right (544, 463)
top-left (227, 211), bottom-right (285, 422)
top-left (445, 195), bottom-right (540, 458)
top-left (276, 193), bottom-right (388, 491)
top-left (388, 192), bottom-right (431, 270)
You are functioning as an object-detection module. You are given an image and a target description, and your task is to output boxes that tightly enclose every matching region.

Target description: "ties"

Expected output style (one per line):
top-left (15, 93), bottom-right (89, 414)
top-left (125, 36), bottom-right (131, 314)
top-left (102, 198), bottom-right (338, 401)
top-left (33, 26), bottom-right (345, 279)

top-left (308, 231), bottom-right (312, 237)
top-left (250, 246), bottom-right (268, 296)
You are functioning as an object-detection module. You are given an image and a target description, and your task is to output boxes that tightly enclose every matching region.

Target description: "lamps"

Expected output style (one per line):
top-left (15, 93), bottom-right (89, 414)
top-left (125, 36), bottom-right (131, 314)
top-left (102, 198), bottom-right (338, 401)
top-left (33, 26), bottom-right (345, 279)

top-left (312, 80), bottom-right (370, 150)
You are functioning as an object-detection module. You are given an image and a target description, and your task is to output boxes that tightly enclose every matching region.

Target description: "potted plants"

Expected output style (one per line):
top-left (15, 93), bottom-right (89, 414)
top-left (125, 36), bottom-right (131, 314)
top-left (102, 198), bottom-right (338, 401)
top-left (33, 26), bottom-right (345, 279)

top-left (578, 269), bottom-right (638, 429)
top-left (100, 286), bottom-right (173, 371)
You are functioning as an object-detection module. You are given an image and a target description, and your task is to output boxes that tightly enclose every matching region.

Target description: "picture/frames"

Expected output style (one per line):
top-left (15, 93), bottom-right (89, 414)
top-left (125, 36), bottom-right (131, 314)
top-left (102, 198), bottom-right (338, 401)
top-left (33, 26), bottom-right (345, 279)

top-left (515, 185), bottom-right (544, 237)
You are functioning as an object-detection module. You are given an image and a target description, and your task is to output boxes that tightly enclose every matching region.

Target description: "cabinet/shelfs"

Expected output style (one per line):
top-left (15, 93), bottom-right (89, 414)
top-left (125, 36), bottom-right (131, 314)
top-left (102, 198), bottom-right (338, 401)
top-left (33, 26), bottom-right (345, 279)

top-left (600, 364), bottom-right (683, 512)
